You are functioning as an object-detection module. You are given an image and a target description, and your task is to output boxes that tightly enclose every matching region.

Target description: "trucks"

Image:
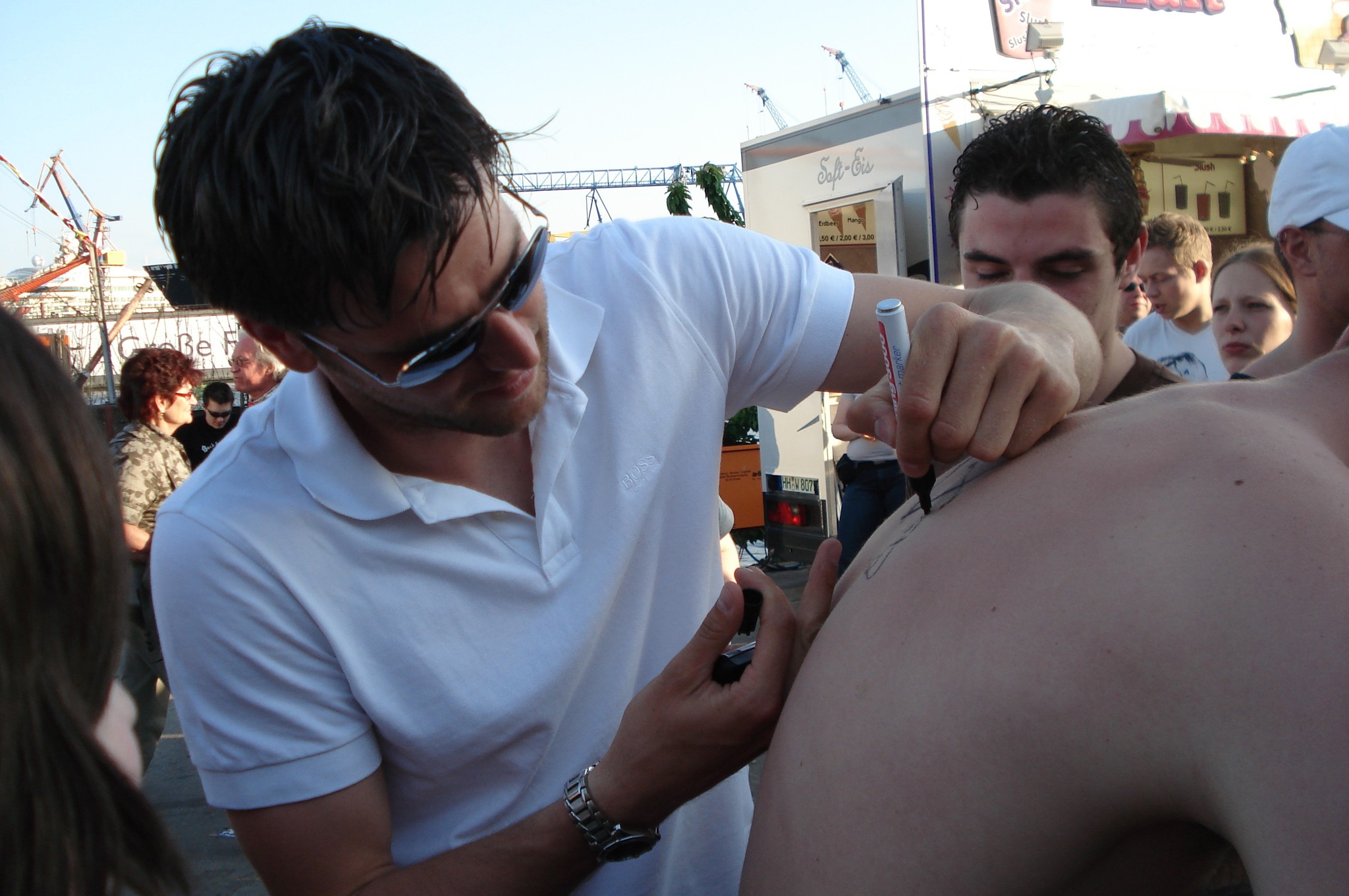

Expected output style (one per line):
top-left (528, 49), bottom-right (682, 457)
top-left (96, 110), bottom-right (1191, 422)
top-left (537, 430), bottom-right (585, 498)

top-left (737, 87), bottom-right (1300, 565)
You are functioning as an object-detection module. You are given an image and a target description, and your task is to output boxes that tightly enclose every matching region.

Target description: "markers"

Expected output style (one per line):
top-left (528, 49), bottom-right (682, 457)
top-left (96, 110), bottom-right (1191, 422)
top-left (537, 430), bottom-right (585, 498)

top-left (873, 298), bottom-right (935, 513)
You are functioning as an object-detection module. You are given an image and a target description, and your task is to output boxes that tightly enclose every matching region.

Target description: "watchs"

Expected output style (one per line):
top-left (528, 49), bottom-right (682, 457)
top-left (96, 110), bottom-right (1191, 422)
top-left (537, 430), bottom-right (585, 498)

top-left (562, 762), bottom-right (662, 862)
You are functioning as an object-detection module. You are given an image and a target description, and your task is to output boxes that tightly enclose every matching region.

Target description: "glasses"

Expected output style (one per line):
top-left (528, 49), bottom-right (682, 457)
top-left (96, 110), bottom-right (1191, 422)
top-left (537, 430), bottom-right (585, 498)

top-left (205, 407), bottom-right (232, 418)
top-left (291, 165), bottom-right (553, 390)
top-left (173, 389), bottom-right (197, 399)
top-left (1123, 282), bottom-right (1145, 292)
top-left (227, 358), bottom-right (259, 367)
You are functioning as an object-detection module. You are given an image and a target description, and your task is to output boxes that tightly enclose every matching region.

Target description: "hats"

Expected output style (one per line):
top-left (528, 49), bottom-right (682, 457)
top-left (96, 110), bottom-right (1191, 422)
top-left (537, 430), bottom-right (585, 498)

top-left (1266, 123), bottom-right (1349, 238)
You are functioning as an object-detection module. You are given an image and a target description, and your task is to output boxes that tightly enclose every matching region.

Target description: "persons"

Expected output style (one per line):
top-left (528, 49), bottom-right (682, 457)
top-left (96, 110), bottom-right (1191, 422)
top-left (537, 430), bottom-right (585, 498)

top-left (147, 22), bottom-right (1102, 896)
top-left (1123, 211), bottom-right (1231, 381)
top-left (734, 326), bottom-right (1348, 896)
top-left (175, 381), bottom-right (237, 469)
top-left (832, 386), bottom-right (908, 583)
top-left (0, 309), bottom-right (185, 896)
top-left (1117, 273), bottom-right (1157, 336)
top-left (1216, 131), bottom-right (1349, 381)
top-left (230, 333), bottom-right (285, 410)
top-left (948, 104), bottom-right (1198, 411)
top-left (101, 348), bottom-right (200, 784)
top-left (1210, 239), bottom-right (1295, 377)
